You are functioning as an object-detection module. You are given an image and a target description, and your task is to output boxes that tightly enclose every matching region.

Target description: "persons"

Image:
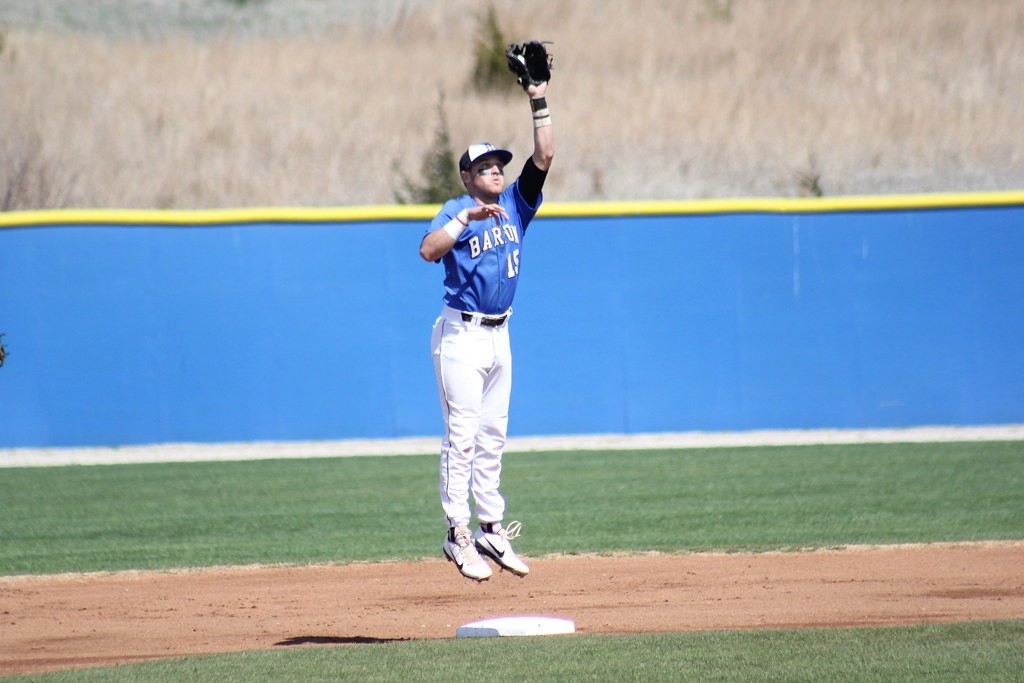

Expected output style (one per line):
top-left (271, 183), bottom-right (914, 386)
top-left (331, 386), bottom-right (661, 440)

top-left (419, 39), bottom-right (555, 582)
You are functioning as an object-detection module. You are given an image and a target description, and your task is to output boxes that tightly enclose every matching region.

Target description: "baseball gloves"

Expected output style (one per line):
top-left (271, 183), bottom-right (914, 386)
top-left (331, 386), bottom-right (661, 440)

top-left (506, 40), bottom-right (551, 91)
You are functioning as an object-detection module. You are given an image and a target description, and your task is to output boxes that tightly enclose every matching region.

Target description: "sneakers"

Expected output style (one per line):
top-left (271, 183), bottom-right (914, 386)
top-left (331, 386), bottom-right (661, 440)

top-left (442, 525), bottom-right (493, 583)
top-left (474, 521), bottom-right (530, 578)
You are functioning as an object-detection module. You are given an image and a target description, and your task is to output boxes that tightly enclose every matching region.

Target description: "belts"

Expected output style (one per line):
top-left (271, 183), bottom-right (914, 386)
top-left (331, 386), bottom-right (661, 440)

top-left (461, 314), bottom-right (507, 327)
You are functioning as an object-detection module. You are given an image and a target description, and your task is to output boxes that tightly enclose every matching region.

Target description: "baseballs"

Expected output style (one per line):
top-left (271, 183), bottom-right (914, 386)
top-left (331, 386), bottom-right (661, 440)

top-left (517, 54), bottom-right (525, 64)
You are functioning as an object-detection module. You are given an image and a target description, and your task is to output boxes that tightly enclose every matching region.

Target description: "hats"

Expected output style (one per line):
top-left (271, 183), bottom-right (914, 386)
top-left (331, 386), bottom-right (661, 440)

top-left (458, 143), bottom-right (513, 185)
